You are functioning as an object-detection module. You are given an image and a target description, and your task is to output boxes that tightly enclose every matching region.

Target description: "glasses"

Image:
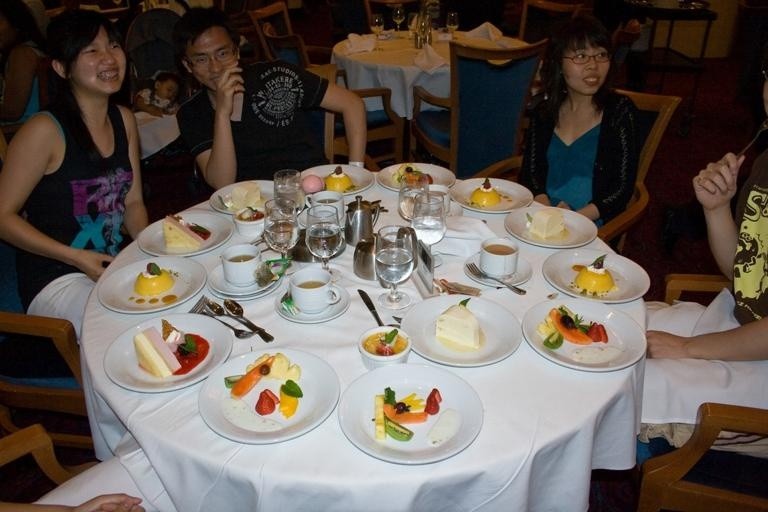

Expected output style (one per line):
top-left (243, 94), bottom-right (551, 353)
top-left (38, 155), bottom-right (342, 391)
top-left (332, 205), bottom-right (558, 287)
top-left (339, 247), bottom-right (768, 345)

top-left (189, 49), bottom-right (237, 66)
top-left (560, 53), bottom-right (612, 64)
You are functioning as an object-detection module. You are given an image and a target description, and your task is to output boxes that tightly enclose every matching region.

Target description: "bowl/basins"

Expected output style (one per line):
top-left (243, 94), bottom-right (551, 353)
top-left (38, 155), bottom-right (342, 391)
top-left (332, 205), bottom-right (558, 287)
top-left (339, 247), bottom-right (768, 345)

top-left (360, 325), bottom-right (412, 371)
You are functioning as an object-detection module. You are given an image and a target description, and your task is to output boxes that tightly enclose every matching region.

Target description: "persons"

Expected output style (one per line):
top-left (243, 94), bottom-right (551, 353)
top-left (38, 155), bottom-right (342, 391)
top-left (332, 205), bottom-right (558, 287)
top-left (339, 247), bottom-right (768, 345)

top-left (132, 72), bottom-right (184, 117)
top-left (0, 6), bottom-right (150, 344)
top-left (0, 494), bottom-right (147, 512)
top-left (640, 68), bottom-right (768, 460)
top-left (169, 5), bottom-right (369, 193)
top-left (516, 14), bottom-right (640, 231)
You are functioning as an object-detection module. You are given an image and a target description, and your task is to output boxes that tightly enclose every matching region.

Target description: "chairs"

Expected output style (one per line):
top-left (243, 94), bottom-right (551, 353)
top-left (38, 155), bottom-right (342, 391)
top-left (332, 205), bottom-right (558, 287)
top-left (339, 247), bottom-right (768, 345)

top-left (1, 2), bottom-right (718, 257)
top-left (636, 271), bottom-right (767, 512)
top-left (2, 258), bottom-right (99, 494)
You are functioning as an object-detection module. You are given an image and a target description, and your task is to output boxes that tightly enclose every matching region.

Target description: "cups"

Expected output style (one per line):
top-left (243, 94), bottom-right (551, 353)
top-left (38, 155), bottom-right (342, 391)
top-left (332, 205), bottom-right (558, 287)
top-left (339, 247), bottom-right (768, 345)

top-left (221, 243), bottom-right (262, 287)
top-left (289, 267), bottom-right (342, 316)
top-left (232, 206), bottom-right (265, 238)
top-left (479, 238), bottom-right (520, 278)
top-left (305, 191), bottom-right (346, 221)
top-left (396, 170), bottom-right (430, 223)
top-left (273, 169), bottom-right (306, 216)
top-left (428, 184), bottom-right (451, 213)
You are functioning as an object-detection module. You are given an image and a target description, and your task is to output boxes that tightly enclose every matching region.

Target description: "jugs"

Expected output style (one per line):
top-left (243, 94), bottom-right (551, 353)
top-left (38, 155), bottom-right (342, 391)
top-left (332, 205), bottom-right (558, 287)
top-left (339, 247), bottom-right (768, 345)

top-left (343, 196), bottom-right (389, 246)
top-left (353, 232), bottom-right (395, 281)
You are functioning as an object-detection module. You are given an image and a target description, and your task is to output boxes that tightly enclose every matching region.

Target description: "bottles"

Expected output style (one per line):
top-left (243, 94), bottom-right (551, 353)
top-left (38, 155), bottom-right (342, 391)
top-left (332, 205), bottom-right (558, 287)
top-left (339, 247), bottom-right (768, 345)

top-left (396, 227), bottom-right (419, 271)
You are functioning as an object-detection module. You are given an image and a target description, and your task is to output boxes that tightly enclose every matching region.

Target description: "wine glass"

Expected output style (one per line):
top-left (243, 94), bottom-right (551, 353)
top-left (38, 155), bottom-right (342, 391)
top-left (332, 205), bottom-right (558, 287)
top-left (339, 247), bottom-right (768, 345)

top-left (262, 198), bottom-right (300, 258)
top-left (375, 225), bottom-right (415, 309)
top-left (366, 4), bottom-right (460, 50)
top-left (411, 192), bottom-right (447, 268)
top-left (305, 205), bottom-right (343, 281)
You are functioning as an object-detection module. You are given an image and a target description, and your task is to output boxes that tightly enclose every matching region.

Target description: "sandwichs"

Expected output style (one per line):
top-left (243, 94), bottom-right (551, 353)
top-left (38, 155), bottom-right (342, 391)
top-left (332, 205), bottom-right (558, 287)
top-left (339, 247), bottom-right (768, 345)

top-left (164, 217), bottom-right (204, 249)
top-left (134, 328), bottom-right (181, 377)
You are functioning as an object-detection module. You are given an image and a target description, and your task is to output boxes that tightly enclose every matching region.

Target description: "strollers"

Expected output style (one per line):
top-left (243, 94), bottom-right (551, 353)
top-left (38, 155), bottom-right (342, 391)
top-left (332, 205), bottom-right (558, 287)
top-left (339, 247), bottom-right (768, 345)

top-left (124, 7), bottom-right (205, 200)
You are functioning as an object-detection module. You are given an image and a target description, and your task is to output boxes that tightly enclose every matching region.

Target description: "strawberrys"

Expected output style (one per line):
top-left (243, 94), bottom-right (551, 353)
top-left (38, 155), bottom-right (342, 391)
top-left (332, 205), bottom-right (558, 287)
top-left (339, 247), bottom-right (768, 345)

top-left (255, 391), bottom-right (275, 415)
top-left (587, 322), bottom-right (601, 341)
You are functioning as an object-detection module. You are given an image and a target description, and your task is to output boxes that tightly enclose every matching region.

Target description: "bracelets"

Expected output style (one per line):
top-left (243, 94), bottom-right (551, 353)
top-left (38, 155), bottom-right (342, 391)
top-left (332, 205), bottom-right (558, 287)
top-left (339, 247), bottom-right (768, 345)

top-left (348, 161), bottom-right (364, 168)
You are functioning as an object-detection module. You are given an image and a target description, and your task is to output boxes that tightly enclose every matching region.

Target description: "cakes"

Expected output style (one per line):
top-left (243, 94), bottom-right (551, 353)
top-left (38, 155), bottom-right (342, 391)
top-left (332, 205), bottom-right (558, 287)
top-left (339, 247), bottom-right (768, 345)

top-left (325, 167), bottom-right (352, 192)
top-left (231, 183), bottom-right (260, 208)
top-left (134, 264), bottom-right (172, 295)
top-left (531, 209), bottom-right (564, 238)
top-left (435, 306), bottom-right (480, 349)
top-left (576, 255), bottom-right (613, 293)
top-left (470, 179), bottom-right (499, 206)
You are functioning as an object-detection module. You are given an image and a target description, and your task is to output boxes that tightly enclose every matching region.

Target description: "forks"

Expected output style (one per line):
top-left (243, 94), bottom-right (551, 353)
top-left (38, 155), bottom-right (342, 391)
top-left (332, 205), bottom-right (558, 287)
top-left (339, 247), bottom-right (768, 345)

top-left (465, 263), bottom-right (528, 296)
top-left (202, 307), bottom-right (264, 340)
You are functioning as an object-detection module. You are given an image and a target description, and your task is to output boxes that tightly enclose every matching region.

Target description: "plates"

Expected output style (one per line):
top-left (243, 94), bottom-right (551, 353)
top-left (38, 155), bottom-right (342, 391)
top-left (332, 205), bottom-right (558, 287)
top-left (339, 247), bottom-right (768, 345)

top-left (443, 202), bottom-right (465, 218)
top-left (97, 255), bottom-right (207, 314)
top-left (462, 251), bottom-right (533, 288)
top-left (207, 257), bottom-right (283, 302)
top-left (450, 177), bottom-right (534, 214)
top-left (298, 163), bottom-right (375, 194)
top-left (274, 283), bottom-right (351, 323)
top-left (521, 297), bottom-right (648, 373)
top-left (542, 249), bottom-right (652, 303)
top-left (504, 206), bottom-right (599, 249)
top-left (102, 313), bottom-right (235, 394)
top-left (198, 344), bottom-right (341, 446)
top-left (399, 294), bottom-right (523, 368)
top-left (337, 361), bottom-right (486, 466)
top-left (137, 210), bottom-right (232, 258)
top-left (210, 179), bottom-right (276, 216)
top-left (377, 163), bottom-right (457, 192)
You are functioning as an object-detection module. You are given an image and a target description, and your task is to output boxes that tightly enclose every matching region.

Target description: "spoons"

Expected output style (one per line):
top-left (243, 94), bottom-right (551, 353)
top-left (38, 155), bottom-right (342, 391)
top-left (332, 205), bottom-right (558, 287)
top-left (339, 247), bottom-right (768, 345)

top-left (203, 299), bottom-right (271, 344)
top-left (223, 299), bottom-right (276, 343)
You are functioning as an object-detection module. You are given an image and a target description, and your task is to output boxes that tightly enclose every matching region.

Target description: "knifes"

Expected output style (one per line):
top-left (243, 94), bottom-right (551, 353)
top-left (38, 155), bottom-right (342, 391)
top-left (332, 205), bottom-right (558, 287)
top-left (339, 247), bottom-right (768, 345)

top-left (356, 289), bottom-right (385, 328)
